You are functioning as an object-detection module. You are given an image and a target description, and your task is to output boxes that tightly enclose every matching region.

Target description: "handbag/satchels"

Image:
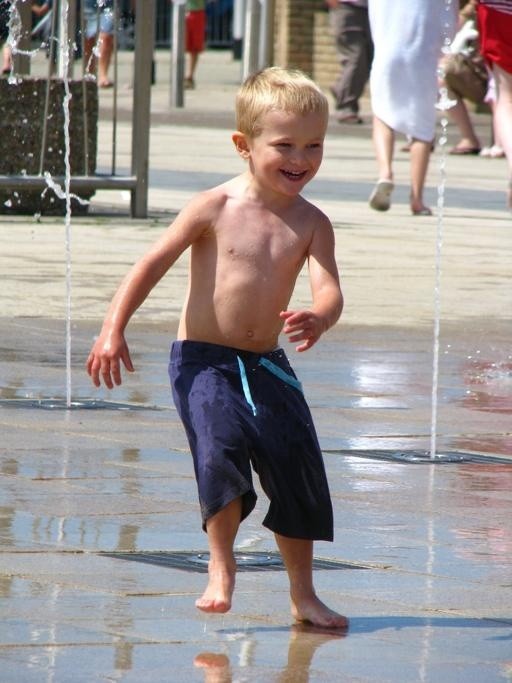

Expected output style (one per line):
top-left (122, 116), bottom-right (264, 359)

top-left (443, 20), bottom-right (489, 100)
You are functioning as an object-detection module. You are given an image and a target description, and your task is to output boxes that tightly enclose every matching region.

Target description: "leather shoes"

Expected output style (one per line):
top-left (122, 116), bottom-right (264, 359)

top-left (338, 115), bottom-right (362, 124)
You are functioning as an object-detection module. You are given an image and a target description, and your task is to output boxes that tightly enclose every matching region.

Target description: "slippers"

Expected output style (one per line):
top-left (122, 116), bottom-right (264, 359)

top-left (368, 179), bottom-right (394, 212)
top-left (413, 208), bottom-right (432, 216)
top-left (449, 146), bottom-right (480, 154)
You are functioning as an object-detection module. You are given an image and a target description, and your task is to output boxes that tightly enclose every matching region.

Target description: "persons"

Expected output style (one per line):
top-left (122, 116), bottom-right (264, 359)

top-left (86, 67), bottom-right (349, 628)
top-left (0, 0), bottom-right (206, 89)
top-left (194, 629), bottom-right (349, 683)
top-left (335, 0), bottom-right (512, 217)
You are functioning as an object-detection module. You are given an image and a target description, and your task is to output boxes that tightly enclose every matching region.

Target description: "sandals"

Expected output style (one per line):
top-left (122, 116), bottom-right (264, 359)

top-left (481, 146), bottom-right (503, 157)
